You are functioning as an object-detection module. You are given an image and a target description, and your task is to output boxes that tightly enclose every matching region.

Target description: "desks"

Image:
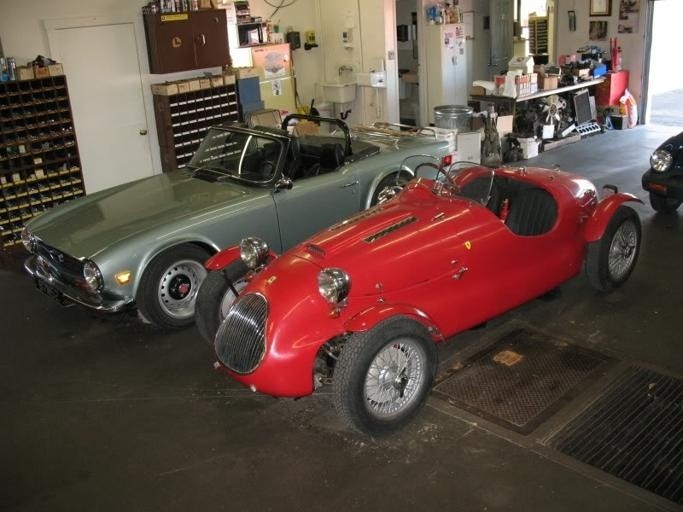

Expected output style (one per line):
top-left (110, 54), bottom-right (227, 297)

top-left (468, 76), bottom-right (605, 133)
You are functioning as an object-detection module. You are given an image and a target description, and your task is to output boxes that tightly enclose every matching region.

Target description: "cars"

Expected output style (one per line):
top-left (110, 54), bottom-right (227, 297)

top-left (19, 111), bottom-right (460, 331)
top-left (193, 155), bottom-right (646, 439)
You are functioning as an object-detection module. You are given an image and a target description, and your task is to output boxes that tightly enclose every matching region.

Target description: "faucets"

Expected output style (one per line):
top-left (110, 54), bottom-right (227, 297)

top-left (339, 65), bottom-right (352, 76)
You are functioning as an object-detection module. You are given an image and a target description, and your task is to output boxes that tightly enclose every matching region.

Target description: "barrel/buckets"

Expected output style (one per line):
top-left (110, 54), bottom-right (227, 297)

top-left (434, 104), bottom-right (473, 133)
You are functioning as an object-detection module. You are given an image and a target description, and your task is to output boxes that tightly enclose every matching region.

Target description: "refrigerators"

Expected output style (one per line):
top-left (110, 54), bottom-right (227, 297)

top-left (425, 23), bottom-right (467, 126)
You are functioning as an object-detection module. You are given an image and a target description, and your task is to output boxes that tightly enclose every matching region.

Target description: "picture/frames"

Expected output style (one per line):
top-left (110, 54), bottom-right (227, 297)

top-left (589, 0), bottom-right (612, 16)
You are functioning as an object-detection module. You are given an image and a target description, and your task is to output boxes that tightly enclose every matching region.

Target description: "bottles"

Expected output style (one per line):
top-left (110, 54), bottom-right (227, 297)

top-left (150, 0), bottom-right (198, 14)
top-left (7, 57), bottom-right (16, 80)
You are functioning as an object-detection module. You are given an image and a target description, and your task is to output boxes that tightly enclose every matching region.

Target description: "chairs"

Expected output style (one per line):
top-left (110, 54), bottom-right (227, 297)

top-left (305, 143), bottom-right (346, 178)
top-left (459, 175), bottom-right (511, 212)
top-left (509, 186), bottom-right (557, 235)
top-left (263, 134), bottom-right (302, 178)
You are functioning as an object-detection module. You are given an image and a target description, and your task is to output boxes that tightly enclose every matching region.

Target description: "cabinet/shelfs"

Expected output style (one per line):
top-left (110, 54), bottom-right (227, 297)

top-left (0, 75), bottom-right (86, 250)
top-left (153, 84), bottom-right (239, 172)
top-left (143, 9), bottom-right (230, 74)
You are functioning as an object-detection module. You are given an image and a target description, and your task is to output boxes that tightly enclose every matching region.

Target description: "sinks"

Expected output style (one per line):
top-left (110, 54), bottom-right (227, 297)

top-left (320, 83), bottom-right (356, 103)
